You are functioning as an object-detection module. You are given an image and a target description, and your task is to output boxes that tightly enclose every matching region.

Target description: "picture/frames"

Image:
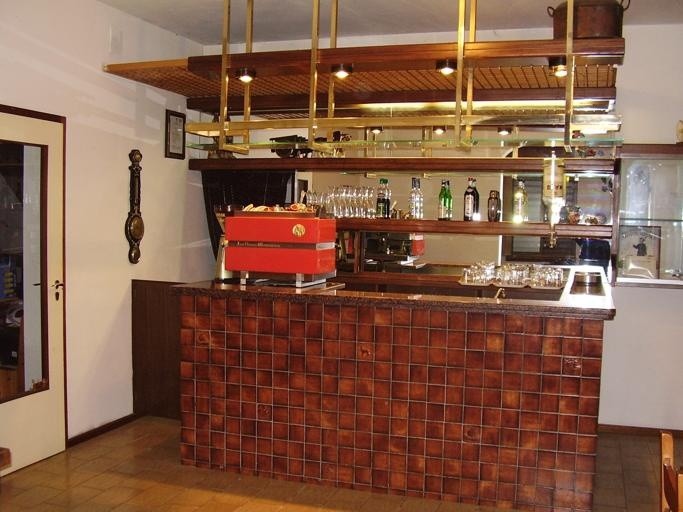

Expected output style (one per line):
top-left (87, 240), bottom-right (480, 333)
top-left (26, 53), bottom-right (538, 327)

top-left (164, 108), bottom-right (186, 160)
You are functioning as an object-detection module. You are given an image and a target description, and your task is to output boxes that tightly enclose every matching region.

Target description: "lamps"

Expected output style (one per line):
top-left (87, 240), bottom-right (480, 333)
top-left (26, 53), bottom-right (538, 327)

top-left (186, 53), bottom-right (625, 85)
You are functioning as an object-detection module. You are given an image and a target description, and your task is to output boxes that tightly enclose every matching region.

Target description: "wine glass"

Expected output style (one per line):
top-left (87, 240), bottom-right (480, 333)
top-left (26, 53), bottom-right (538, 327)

top-left (304, 186), bottom-right (375, 220)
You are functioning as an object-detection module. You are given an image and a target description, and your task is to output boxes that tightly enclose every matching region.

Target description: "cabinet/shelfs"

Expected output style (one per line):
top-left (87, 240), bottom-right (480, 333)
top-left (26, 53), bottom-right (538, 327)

top-left (190, 157), bottom-right (619, 292)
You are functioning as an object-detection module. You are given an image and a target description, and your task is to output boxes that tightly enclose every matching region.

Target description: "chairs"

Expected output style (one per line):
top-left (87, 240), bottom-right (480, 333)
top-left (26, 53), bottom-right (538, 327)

top-left (658, 430), bottom-right (683, 511)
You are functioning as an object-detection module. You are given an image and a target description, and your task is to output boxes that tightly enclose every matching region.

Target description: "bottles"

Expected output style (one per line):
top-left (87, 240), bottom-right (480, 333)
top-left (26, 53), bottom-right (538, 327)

top-left (512, 177), bottom-right (530, 223)
top-left (463, 177), bottom-right (481, 221)
top-left (376, 178), bottom-right (392, 219)
top-left (437, 179), bottom-right (454, 221)
top-left (407, 175), bottom-right (425, 220)
top-left (487, 188), bottom-right (503, 223)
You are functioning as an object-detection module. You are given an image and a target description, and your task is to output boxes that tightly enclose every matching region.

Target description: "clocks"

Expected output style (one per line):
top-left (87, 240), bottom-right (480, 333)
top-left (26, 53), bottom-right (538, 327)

top-left (125, 149), bottom-right (145, 264)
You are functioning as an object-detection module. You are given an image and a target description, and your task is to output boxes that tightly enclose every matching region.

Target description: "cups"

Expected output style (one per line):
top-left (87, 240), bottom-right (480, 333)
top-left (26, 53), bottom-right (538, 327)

top-left (458, 259), bottom-right (563, 287)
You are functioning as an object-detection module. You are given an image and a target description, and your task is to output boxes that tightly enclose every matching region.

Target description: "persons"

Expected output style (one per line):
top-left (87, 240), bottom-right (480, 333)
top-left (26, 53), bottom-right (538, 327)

top-left (573, 216), bottom-right (610, 278)
top-left (633, 237), bottom-right (647, 256)
